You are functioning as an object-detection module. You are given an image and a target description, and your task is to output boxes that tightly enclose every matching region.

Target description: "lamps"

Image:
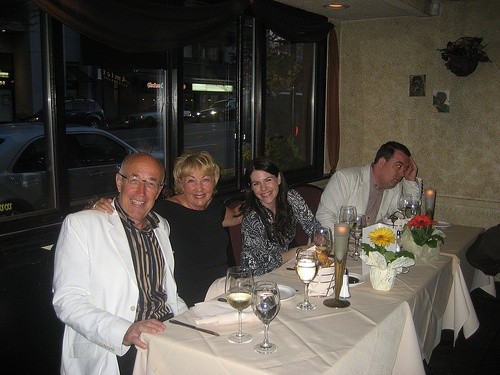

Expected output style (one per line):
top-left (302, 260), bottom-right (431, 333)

top-left (424, 189), bottom-right (436, 221)
top-left (323, 222), bottom-right (351, 307)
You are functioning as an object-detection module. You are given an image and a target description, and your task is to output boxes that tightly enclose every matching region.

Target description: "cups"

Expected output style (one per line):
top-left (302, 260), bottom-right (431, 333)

top-left (314, 226), bottom-right (332, 256)
top-left (395, 192), bottom-right (422, 219)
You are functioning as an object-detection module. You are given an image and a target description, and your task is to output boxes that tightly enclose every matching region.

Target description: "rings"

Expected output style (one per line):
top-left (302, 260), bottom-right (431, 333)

top-left (93, 203), bottom-right (97, 208)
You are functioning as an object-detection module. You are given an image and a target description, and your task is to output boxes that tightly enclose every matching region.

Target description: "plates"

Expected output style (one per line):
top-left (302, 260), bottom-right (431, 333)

top-left (382, 220), bottom-right (408, 226)
top-left (348, 272), bottom-right (366, 288)
top-left (435, 221), bottom-right (450, 228)
top-left (277, 284), bottom-right (297, 302)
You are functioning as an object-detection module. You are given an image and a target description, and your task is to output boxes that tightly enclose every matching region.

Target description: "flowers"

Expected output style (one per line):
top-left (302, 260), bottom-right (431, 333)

top-left (357, 228), bottom-right (415, 271)
top-left (406, 216), bottom-right (445, 249)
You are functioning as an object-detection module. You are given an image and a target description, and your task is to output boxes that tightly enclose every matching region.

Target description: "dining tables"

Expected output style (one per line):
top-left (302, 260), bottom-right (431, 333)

top-left (131, 243), bottom-right (481, 375)
top-left (434, 224), bottom-right (485, 265)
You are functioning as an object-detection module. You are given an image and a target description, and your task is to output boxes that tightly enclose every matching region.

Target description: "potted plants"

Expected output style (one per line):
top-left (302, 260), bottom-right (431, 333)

top-left (437, 36), bottom-right (493, 77)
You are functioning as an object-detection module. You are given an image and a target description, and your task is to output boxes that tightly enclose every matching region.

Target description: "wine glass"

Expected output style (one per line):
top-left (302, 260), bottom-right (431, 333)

top-left (250, 280), bottom-right (282, 354)
top-left (295, 248), bottom-right (319, 312)
top-left (224, 265), bottom-right (254, 345)
top-left (339, 205), bottom-right (366, 261)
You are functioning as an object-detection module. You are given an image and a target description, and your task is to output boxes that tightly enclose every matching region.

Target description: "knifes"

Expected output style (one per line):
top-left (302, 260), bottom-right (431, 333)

top-left (169, 319), bottom-right (220, 337)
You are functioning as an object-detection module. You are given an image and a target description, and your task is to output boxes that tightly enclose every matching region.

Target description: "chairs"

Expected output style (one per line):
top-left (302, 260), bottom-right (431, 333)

top-left (225, 183), bottom-right (500, 375)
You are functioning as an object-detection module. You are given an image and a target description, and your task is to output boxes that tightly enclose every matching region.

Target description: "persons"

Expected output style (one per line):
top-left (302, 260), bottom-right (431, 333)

top-left (315, 142), bottom-right (422, 240)
top-left (240, 157), bottom-right (327, 277)
top-left (52, 154), bottom-right (187, 375)
top-left (90, 151), bottom-right (244, 310)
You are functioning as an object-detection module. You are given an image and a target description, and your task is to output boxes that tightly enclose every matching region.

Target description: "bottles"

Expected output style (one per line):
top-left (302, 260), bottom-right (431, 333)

top-left (339, 268), bottom-right (351, 299)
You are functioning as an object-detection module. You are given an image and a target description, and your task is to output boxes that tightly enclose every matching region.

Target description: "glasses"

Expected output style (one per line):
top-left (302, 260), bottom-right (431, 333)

top-left (120, 173), bottom-right (163, 191)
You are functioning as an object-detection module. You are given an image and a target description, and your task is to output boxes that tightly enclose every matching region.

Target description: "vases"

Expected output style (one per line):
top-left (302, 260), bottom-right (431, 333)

top-left (370, 266), bottom-right (395, 291)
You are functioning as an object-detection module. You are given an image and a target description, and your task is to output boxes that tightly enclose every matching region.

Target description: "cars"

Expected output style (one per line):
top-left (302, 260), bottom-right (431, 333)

top-left (0, 127), bottom-right (164, 219)
top-left (200, 99), bottom-right (238, 122)
top-left (34, 98), bottom-right (104, 128)
top-left (121, 106), bottom-right (158, 129)
